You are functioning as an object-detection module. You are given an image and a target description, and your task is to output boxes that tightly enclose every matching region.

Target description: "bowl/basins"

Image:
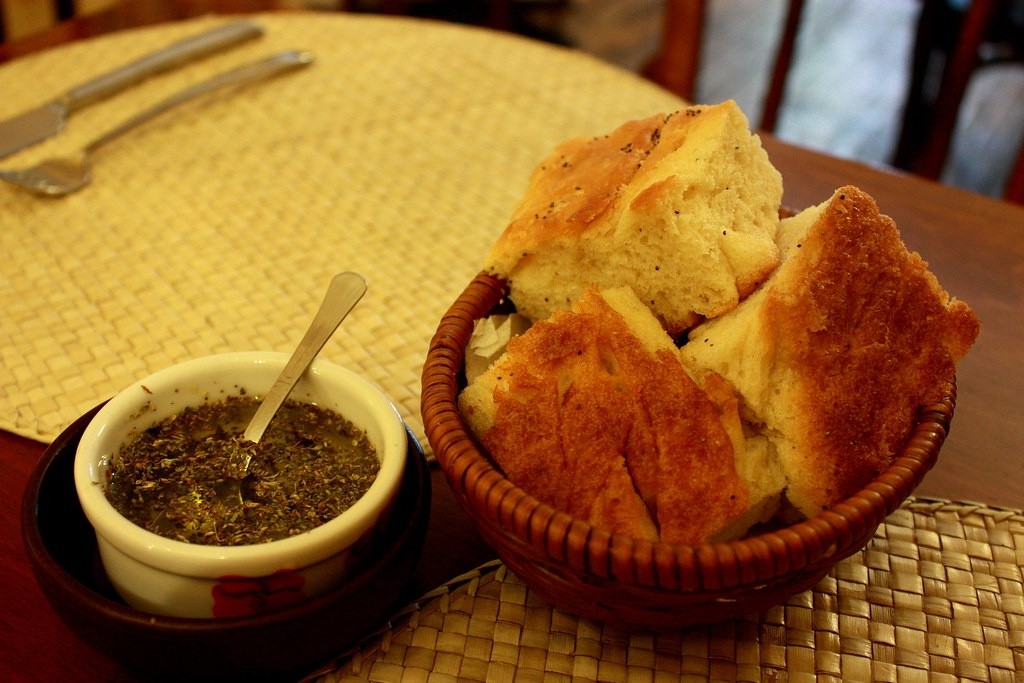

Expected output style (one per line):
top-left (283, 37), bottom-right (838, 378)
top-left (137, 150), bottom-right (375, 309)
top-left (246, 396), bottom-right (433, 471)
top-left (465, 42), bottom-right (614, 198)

top-left (18, 394), bottom-right (435, 673)
top-left (73, 350), bottom-right (408, 619)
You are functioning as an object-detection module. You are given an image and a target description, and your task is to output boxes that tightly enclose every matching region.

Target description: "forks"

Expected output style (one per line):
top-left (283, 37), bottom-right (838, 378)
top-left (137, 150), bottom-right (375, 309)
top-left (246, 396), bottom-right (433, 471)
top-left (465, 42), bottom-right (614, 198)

top-left (0, 50), bottom-right (313, 197)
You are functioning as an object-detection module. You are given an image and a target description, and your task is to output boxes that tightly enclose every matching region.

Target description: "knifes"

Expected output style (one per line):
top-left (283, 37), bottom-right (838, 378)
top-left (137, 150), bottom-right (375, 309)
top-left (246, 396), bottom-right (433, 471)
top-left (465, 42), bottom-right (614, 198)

top-left (0, 24), bottom-right (263, 157)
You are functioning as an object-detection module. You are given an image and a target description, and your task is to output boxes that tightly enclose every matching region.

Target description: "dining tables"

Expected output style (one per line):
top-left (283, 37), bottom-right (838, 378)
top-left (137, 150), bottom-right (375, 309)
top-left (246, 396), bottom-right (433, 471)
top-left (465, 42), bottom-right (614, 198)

top-left (1, 12), bottom-right (1024, 683)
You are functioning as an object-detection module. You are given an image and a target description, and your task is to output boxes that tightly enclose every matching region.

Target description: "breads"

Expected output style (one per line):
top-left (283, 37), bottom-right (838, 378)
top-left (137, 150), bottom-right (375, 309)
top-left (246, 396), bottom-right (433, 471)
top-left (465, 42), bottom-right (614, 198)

top-left (457, 98), bottom-right (982, 548)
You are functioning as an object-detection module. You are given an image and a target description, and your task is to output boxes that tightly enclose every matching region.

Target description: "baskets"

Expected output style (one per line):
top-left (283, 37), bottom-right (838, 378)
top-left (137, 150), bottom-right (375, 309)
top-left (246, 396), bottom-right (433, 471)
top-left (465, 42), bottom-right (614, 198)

top-left (421, 204), bottom-right (957, 635)
